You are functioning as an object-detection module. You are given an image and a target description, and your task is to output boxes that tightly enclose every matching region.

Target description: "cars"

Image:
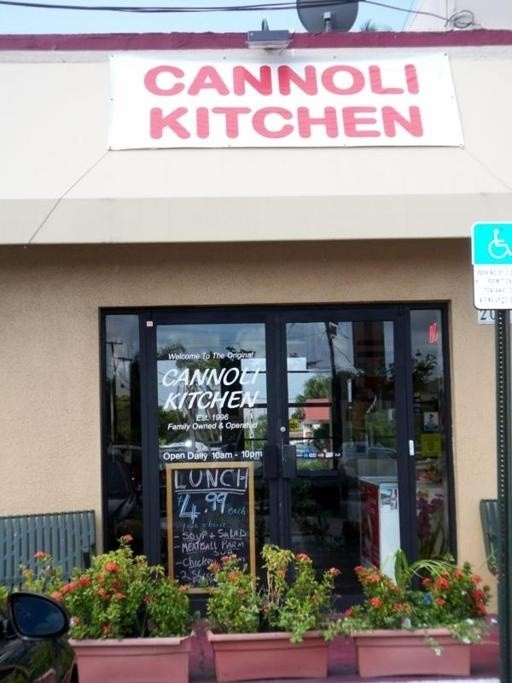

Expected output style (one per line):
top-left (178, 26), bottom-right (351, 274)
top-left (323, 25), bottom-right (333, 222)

top-left (0, 587), bottom-right (81, 683)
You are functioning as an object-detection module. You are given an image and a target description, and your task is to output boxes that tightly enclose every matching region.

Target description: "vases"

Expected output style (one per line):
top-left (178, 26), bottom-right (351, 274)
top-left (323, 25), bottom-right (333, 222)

top-left (68, 634), bottom-right (190, 683)
top-left (208, 626), bottom-right (330, 682)
top-left (349, 625), bottom-right (473, 679)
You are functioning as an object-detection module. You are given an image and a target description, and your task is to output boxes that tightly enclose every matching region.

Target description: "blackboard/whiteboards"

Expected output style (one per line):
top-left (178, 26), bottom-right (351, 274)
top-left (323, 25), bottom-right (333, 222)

top-left (165, 461), bottom-right (256, 594)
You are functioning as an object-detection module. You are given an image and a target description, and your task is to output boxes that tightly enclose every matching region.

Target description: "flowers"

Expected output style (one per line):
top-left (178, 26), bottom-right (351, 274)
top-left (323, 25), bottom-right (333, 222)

top-left (64, 535), bottom-right (194, 639)
top-left (344, 553), bottom-right (490, 640)
top-left (205, 545), bottom-right (340, 637)
